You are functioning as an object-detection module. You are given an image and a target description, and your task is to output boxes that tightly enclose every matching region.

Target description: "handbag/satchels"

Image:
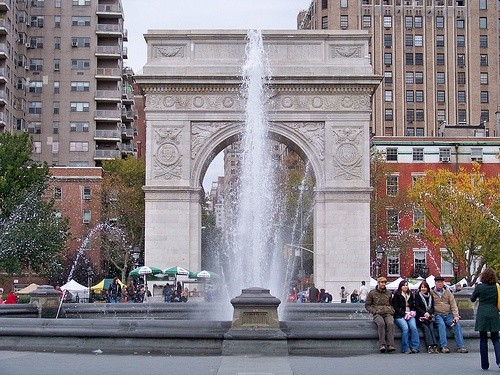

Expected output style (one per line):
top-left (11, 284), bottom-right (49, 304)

top-left (496, 283), bottom-right (500, 312)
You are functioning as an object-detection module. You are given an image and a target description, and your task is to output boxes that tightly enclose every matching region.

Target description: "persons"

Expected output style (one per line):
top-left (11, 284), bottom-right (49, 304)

top-left (365, 276), bottom-right (469, 352)
top-left (287, 282), bottom-right (333, 303)
top-left (470, 267), bottom-right (500, 370)
top-left (163, 281), bottom-right (216, 303)
top-left (350, 280), bottom-right (369, 302)
top-left (339, 285), bottom-right (350, 303)
top-left (0, 289), bottom-right (20, 304)
top-left (106, 277), bottom-right (152, 303)
top-left (56, 284), bottom-right (65, 303)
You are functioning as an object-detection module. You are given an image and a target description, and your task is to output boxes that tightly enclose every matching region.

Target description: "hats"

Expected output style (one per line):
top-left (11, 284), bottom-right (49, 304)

top-left (377, 277), bottom-right (387, 281)
top-left (434, 277), bottom-right (444, 281)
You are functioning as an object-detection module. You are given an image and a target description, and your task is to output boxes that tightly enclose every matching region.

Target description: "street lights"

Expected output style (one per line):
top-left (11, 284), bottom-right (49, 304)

top-left (86, 266), bottom-right (94, 303)
top-left (375, 247), bottom-right (385, 277)
top-left (420, 266), bottom-right (430, 282)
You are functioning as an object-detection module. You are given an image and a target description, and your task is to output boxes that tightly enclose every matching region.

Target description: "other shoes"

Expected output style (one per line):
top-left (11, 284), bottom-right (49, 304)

top-left (481, 366), bottom-right (488, 371)
top-left (440, 347), bottom-right (450, 352)
top-left (426, 347), bottom-right (440, 353)
top-left (402, 349), bottom-right (419, 354)
top-left (497, 363), bottom-right (500, 368)
top-left (379, 345), bottom-right (396, 353)
top-left (457, 347), bottom-right (469, 353)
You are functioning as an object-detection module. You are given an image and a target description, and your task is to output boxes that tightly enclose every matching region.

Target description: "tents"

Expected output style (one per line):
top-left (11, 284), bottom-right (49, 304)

top-left (15, 283), bottom-right (39, 295)
top-left (368, 274), bottom-right (468, 292)
top-left (90, 279), bottom-right (127, 294)
top-left (60, 279), bottom-right (89, 303)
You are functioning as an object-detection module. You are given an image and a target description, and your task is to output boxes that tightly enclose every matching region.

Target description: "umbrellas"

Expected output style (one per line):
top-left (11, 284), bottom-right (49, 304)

top-left (129, 266), bottom-right (162, 291)
top-left (188, 269), bottom-right (220, 281)
top-left (162, 266), bottom-right (193, 290)
